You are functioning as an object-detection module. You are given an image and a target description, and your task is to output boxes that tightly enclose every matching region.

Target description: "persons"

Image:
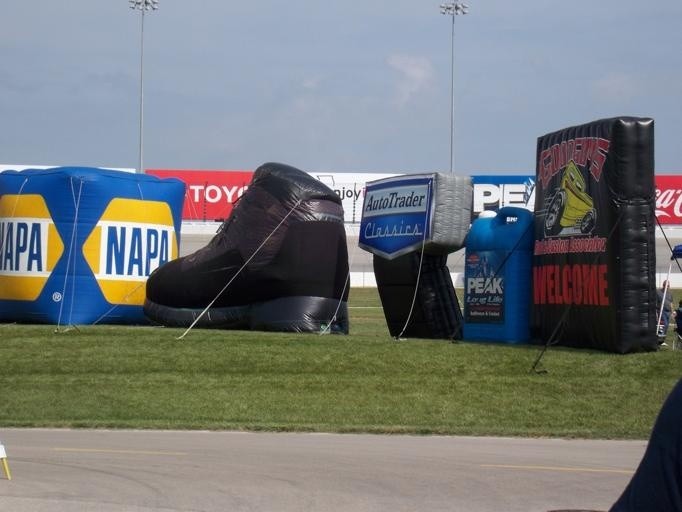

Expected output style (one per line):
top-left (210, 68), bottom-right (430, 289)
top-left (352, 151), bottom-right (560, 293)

top-left (657, 280), bottom-right (675, 347)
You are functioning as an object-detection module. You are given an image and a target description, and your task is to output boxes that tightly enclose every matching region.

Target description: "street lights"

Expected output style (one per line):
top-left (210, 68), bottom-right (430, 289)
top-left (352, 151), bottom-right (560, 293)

top-left (440, 0), bottom-right (469, 173)
top-left (128, 0), bottom-right (160, 174)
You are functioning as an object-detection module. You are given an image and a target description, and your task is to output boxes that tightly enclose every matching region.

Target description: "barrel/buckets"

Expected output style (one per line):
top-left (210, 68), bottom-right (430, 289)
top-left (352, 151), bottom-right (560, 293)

top-left (462, 209), bottom-right (531, 346)
